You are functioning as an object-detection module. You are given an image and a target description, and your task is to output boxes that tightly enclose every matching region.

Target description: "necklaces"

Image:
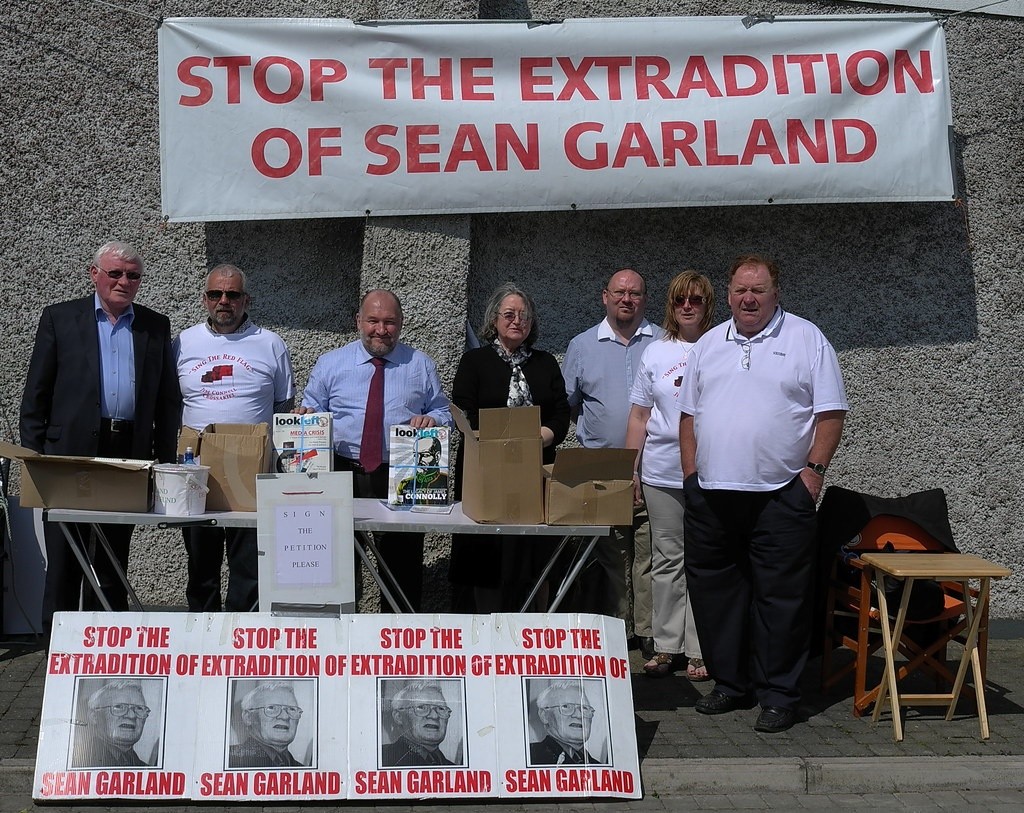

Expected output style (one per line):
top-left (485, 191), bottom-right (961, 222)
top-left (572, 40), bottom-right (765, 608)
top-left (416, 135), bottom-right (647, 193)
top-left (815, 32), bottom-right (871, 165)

top-left (681, 343), bottom-right (696, 359)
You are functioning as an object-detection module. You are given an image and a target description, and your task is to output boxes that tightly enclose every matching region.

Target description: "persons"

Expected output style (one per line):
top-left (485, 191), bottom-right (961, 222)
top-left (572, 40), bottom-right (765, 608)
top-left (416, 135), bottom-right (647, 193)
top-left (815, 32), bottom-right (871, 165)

top-left (19, 243), bottom-right (183, 670)
top-left (290, 290), bottom-right (456, 613)
top-left (626, 271), bottom-right (714, 682)
top-left (383, 682), bottom-right (456, 766)
top-left (230, 683), bottom-right (306, 767)
top-left (175, 263), bottom-right (296, 612)
top-left (530, 681), bottom-right (603, 764)
top-left (675, 255), bottom-right (850, 731)
top-left (75, 682), bottom-right (151, 767)
top-left (452, 282), bottom-right (570, 613)
top-left (562, 269), bottom-right (668, 660)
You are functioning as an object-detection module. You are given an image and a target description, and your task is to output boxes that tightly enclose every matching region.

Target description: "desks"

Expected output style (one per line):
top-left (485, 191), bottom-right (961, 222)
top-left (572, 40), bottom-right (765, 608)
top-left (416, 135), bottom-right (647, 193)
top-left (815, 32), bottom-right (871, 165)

top-left (42, 506), bottom-right (610, 612)
top-left (861, 552), bottom-right (1011, 744)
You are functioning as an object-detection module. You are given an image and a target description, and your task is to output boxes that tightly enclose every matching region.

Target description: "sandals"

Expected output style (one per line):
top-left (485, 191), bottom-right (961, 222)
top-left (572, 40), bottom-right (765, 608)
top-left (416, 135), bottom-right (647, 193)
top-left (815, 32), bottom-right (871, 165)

top-left (687, 658), bottom-right (713, 679)
top-left (643, 653), bottom-right (675, 674)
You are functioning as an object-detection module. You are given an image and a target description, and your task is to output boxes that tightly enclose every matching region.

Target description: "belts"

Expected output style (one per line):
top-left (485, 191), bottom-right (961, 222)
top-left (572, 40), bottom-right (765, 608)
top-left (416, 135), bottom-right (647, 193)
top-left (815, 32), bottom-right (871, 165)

top-left (333, 454), bottom-right (389, 475)
top-left (102, 418), bottom-right (136, 432)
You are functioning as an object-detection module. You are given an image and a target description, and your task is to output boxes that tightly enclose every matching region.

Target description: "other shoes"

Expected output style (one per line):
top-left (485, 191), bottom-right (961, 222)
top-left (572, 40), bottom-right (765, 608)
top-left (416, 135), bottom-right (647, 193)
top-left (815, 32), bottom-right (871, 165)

top-left (627, 635), bottom-right (641, 650)
top-left (640, 637), bottom-right (660, 659)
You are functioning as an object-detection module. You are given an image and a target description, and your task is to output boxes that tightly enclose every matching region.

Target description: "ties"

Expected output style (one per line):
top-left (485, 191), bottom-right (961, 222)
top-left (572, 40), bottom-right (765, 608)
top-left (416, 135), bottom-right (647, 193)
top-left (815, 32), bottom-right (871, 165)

top-left (359, 357), bottom-right (390, 473)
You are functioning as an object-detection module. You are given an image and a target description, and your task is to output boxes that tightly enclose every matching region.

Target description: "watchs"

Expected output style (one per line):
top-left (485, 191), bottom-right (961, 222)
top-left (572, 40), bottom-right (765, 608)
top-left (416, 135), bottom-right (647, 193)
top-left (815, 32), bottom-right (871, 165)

top-left (807, 462), bottom-right (826, 475)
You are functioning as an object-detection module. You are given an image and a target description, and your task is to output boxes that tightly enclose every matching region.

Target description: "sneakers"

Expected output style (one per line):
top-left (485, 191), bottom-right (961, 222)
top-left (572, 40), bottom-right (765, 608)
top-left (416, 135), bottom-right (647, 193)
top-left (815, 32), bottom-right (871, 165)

top-left (753, 706), bottom-right (797, 732)
top-left (695, 689), bottom-right (757, 714)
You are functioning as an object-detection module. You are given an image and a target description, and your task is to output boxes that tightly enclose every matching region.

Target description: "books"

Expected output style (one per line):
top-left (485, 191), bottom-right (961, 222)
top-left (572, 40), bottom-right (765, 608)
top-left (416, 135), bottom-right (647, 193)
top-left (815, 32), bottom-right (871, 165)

top-left (388, 424), bottom-right (454, 506)
top-left (272, 412), bottom-right (334, 473)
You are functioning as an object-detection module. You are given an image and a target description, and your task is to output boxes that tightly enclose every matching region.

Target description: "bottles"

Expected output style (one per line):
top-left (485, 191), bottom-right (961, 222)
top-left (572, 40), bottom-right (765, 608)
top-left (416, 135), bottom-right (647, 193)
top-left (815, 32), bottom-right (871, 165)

top-left (184, 446), bottom-right (196, 465)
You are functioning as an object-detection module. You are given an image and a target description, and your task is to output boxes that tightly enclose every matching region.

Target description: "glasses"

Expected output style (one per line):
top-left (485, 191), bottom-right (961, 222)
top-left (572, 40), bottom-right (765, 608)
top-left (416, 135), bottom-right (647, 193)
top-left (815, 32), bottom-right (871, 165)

top-left (493, 312), bottom-right (531, 320)
top-left (206, 290), bottom-right (246, 299)
top-left (608, 289), bottom-right (644, 299)
top-left (399, 704), bottom-right (452, 720)
top-left (545, 703), bottom-right (595, 719)
top-left (248, 705), bottom-right (303, 720)
top-left (673, 295), bottom-right (708, 306)
top-left (94, 703), bottom-right (152, 719)
top-left (94, 264), bottom-right (141, 280)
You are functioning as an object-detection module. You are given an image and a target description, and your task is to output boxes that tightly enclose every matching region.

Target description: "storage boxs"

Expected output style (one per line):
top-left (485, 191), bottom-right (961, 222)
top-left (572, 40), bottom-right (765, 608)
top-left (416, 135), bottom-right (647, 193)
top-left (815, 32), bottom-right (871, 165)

top-left (449, 403), bottom-right (545, 524)
top-left (541, 449), bottom-right (636, 525)
top-left (180, 422), bottom-right (268, 511)
top-left (0, 441), bottom-right (158, 514)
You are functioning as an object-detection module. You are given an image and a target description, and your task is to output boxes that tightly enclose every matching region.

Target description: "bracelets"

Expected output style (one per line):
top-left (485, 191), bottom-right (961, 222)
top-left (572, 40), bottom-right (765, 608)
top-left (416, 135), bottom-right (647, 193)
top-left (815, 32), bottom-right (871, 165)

top-left (633, 471), bottom-right (638, 475)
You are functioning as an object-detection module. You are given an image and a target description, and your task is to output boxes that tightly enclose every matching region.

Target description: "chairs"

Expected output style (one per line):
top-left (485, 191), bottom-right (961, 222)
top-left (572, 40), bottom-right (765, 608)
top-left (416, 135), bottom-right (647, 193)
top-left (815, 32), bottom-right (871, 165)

top-left (824, 485), bottom-right (990, 717)
